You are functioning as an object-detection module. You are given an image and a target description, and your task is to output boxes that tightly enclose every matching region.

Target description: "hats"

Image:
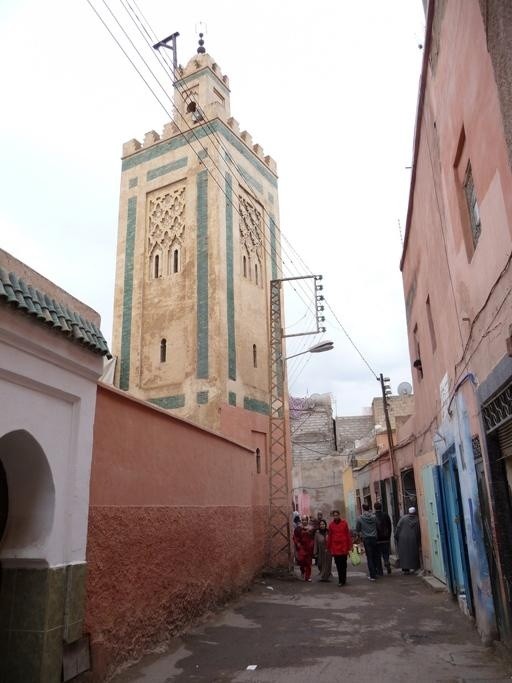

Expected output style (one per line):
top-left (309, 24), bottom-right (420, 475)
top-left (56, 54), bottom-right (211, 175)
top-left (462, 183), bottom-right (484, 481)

top-left (409, 507), bottom-right (416, 514)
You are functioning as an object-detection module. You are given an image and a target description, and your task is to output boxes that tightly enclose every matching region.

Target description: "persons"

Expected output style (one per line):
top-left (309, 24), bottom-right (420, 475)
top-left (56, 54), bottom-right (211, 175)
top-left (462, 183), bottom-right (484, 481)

top-left (393, 507), bottom-right (420, 576)
top-left (355, 503), bottom-right (383, 582)
top-left (371, 502), bottom-right (395, 573)
top-left (293, 507), bottom-right (353, 587)
